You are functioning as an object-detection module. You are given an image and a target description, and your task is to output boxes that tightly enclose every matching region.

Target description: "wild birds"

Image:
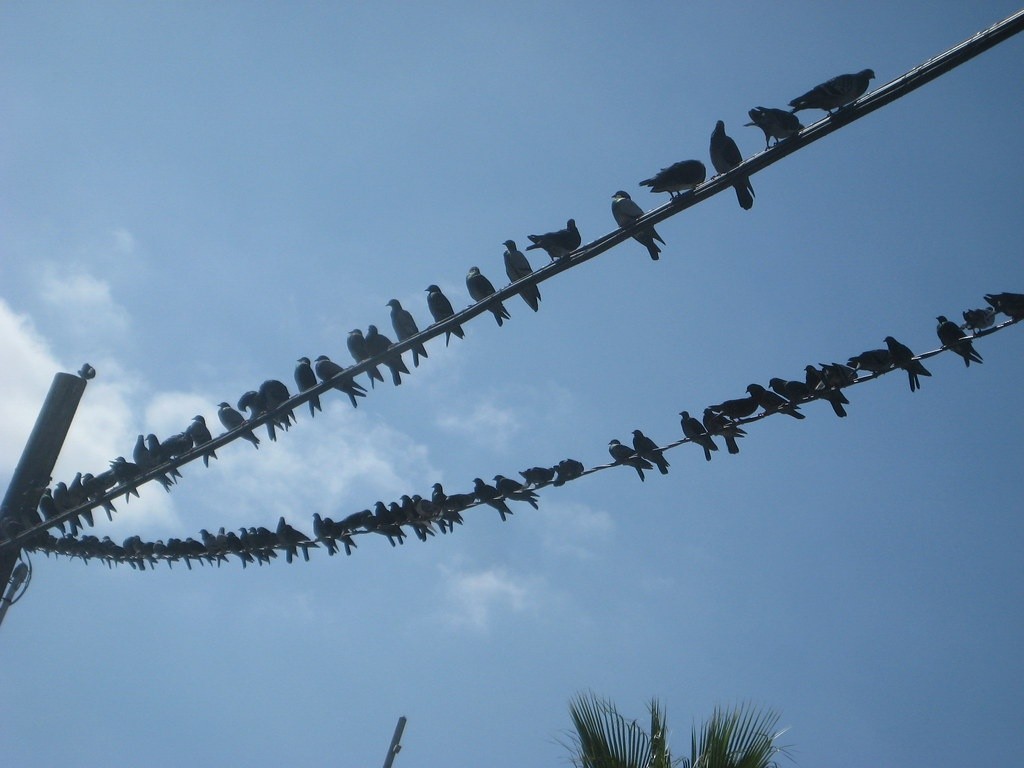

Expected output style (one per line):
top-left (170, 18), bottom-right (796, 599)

top-left (18, 67), bottom-right (1024, 571)
